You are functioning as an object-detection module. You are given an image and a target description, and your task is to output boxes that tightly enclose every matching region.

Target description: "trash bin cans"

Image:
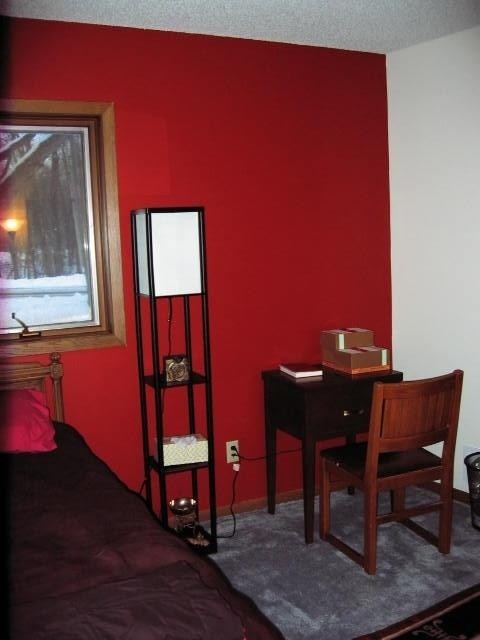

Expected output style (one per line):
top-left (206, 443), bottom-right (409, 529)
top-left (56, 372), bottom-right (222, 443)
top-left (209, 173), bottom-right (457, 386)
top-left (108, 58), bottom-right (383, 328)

top-left (463, 450), bottom-right (480, 530)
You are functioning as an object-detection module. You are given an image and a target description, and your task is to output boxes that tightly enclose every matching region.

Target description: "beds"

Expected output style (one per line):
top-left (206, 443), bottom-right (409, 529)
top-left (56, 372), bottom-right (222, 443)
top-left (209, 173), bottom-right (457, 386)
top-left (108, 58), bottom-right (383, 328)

top-left (0, 351), bottom-right (282, 639)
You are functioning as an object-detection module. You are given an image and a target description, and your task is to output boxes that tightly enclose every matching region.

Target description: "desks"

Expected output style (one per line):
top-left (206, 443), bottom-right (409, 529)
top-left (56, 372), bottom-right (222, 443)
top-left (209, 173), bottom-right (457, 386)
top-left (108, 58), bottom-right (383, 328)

top-left (264, 366), bottom-right (405, 545)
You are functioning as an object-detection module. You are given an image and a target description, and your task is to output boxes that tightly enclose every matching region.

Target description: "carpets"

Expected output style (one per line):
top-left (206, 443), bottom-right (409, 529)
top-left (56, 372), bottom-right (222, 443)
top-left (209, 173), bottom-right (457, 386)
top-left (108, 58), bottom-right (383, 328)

top-left (177, 484), bottom-right (478, 640)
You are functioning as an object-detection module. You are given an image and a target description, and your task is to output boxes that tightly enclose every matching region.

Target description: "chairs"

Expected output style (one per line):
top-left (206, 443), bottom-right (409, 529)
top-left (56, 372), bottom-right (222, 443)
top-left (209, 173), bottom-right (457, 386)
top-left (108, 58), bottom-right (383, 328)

top-left (319, 368), bottom-right (466, 575)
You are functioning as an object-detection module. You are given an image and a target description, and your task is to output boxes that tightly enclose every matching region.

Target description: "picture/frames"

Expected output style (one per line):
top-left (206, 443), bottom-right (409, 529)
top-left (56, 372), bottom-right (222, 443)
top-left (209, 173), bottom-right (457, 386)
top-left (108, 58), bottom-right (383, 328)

top-left (0, 95), bottom-right (129, 359)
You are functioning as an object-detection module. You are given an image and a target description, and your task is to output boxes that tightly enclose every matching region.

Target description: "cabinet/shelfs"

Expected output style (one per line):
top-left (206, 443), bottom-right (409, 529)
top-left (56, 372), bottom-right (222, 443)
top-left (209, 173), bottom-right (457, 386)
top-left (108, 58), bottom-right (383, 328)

top-left (131, 205), bottom-right (219, 555)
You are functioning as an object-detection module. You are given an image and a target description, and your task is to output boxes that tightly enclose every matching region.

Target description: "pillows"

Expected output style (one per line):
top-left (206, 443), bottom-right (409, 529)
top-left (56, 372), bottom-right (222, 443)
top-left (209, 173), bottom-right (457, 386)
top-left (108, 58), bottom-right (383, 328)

top-left (1, 388), bottom-right (58, 455)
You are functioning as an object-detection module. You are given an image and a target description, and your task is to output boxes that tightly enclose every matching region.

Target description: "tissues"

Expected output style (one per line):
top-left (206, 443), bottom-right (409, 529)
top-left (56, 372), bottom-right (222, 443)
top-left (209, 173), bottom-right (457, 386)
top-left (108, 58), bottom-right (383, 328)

top-left (155, 433), bottom-right (208, 467)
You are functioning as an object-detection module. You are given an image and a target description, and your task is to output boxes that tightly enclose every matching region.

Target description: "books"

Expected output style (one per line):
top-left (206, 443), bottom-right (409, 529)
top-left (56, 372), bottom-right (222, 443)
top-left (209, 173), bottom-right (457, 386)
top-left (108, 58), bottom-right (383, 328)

top-left (279, 363), bottom-right (324, 378)
